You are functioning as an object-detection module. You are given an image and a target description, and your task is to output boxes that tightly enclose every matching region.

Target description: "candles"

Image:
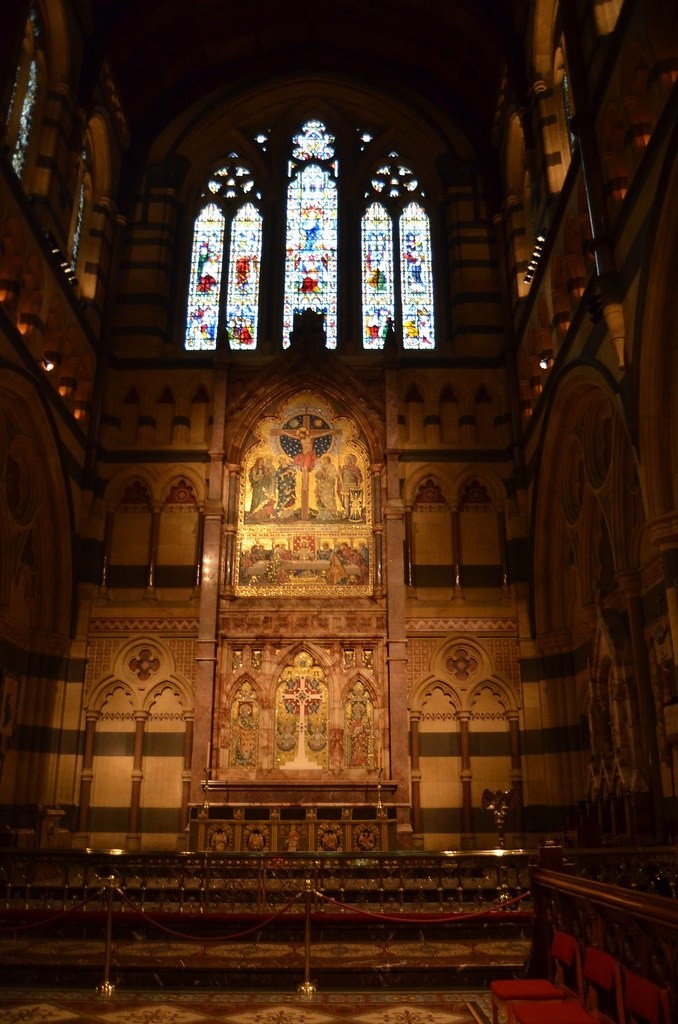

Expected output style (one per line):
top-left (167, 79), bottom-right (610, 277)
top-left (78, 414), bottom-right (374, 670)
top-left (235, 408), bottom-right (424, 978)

top-left (377, 743), bottom-right (382, 769)
top-left (206, 741), bottom-right (210, 769)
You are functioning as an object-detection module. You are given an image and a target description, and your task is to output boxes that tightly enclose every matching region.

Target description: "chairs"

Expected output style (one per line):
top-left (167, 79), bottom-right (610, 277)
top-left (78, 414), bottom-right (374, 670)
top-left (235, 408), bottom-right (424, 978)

top-left (490, 930), bottom-right (584, 1024)
top-left (506, 946), bottom-right (626, 1024)
top-left (623, 969), bottom-right (670, 1024)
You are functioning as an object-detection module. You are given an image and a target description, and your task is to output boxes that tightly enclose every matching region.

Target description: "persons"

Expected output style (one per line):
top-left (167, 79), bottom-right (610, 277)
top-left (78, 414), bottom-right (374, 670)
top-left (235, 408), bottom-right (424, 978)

top-left (491, 788), bottom-right (507, 823)
